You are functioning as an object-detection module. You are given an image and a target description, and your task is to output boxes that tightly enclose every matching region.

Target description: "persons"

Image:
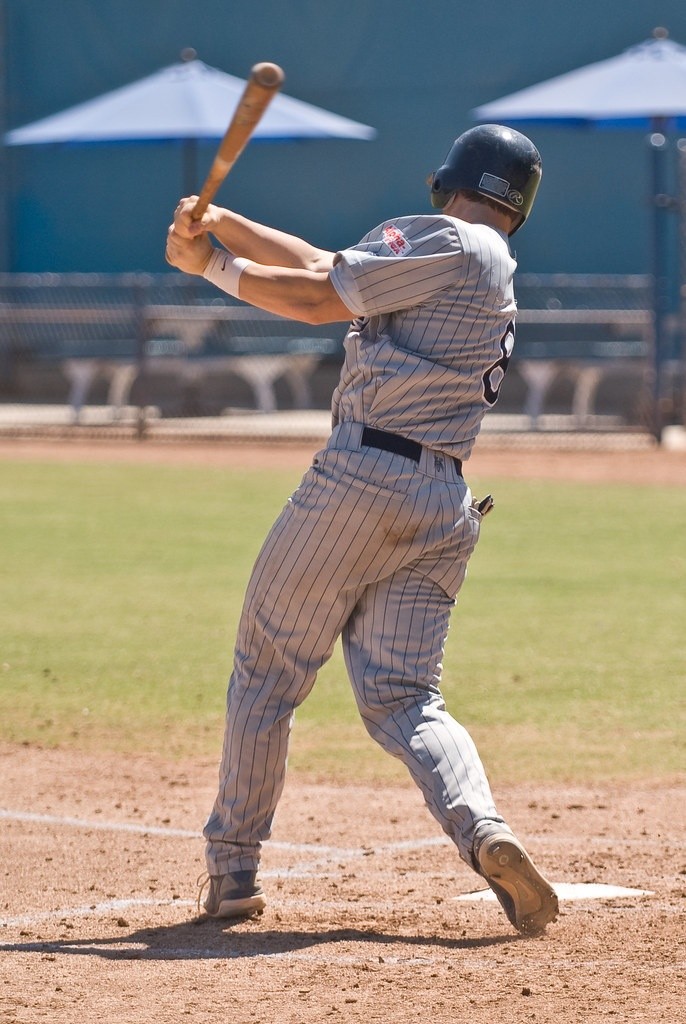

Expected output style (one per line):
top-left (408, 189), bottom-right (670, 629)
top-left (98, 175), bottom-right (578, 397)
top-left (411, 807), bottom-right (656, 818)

top-left (165, 124), bottom-right (559, 932)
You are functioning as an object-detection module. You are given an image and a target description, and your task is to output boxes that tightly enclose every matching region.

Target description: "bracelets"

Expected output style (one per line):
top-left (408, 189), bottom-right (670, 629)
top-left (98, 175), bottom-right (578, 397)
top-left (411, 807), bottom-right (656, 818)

top-left (203, 247), bottom-right (252, 300)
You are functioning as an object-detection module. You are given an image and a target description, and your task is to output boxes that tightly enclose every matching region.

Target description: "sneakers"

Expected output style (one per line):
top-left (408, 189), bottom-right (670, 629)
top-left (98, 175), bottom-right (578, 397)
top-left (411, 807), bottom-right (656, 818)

top-left (196, 868), bottom-right (267, 917)
top-left (470, 822), bottom-right (559, 935)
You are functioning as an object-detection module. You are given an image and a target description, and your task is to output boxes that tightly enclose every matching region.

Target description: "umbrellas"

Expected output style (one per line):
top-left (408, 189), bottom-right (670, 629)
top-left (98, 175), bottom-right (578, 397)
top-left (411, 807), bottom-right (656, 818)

top-left (6, 59), bottom-right (379, 196)
top-left (478, 35), bottom-right (686, 447)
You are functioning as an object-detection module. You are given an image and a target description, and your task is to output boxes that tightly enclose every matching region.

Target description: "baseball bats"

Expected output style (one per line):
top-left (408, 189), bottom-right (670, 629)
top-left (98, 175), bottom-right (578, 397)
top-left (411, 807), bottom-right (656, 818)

top-left (188, 58), bottom-right (287, 239)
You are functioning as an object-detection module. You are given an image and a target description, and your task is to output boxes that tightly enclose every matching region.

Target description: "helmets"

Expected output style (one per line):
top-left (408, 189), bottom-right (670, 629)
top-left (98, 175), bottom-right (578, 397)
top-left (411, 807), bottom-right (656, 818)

top-left (430, 124), bottom-right (543, 237)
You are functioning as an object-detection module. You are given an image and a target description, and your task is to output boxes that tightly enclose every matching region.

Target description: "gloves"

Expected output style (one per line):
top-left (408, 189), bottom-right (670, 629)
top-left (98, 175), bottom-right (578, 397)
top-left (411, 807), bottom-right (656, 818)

top-left (472, 494), bottom-right (495, 516)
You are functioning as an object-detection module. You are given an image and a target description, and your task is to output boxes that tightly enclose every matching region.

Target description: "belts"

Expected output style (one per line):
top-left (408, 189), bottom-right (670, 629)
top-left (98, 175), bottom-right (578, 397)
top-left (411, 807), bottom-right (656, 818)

top-left (361, 426), bottom-right (463, 478)
